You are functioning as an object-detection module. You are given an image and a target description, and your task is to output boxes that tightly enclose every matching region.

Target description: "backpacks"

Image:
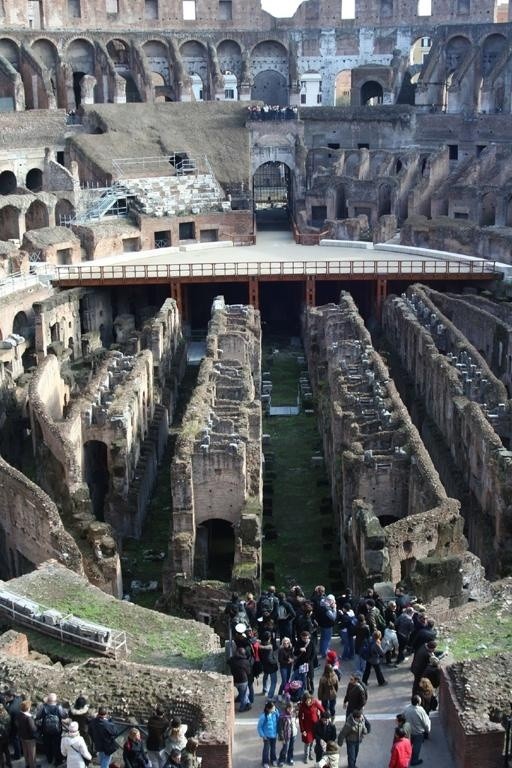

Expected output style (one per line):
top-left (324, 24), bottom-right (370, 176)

top-left (277, 605), bottom-right (287, 620)
top-left (349, 618), bottom-right (359, 636)
top-left (360, 638), bottom-right (371, 660)
top-left (260, 597), bottom-right (274, 617)
top-left (430, 692), bottom-right (438, 711)
top-left (375, 614), bottom-right (387, 633)
top-left (43, 713), bottom-right (62, 738)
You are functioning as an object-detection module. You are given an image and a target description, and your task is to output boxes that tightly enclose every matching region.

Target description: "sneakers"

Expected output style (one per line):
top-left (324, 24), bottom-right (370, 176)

top-left (411, 758), bottom-right (425, 766)
top-left (239, 703), bottom-right (251, 712)
top-left (263, 755), bottom-right (313, 768)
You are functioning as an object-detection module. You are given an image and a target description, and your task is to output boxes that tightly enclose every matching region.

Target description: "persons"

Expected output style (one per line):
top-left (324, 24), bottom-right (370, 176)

top-left (0, 673), bottom-right (206, 768)
top-left (248, 103), bottom-right (298, 121)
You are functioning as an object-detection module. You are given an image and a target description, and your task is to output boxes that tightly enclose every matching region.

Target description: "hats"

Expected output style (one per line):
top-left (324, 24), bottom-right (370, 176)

top-left (68, 721), bottom-right (79, 732)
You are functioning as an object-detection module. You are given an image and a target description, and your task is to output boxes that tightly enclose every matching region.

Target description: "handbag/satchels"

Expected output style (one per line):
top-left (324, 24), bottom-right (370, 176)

top-left (252, 660), bottom-right (262, 677)
top-left (83, 758), bottom-right (92, 766)
top-left (102, 736), bottom-right (118, 756)
